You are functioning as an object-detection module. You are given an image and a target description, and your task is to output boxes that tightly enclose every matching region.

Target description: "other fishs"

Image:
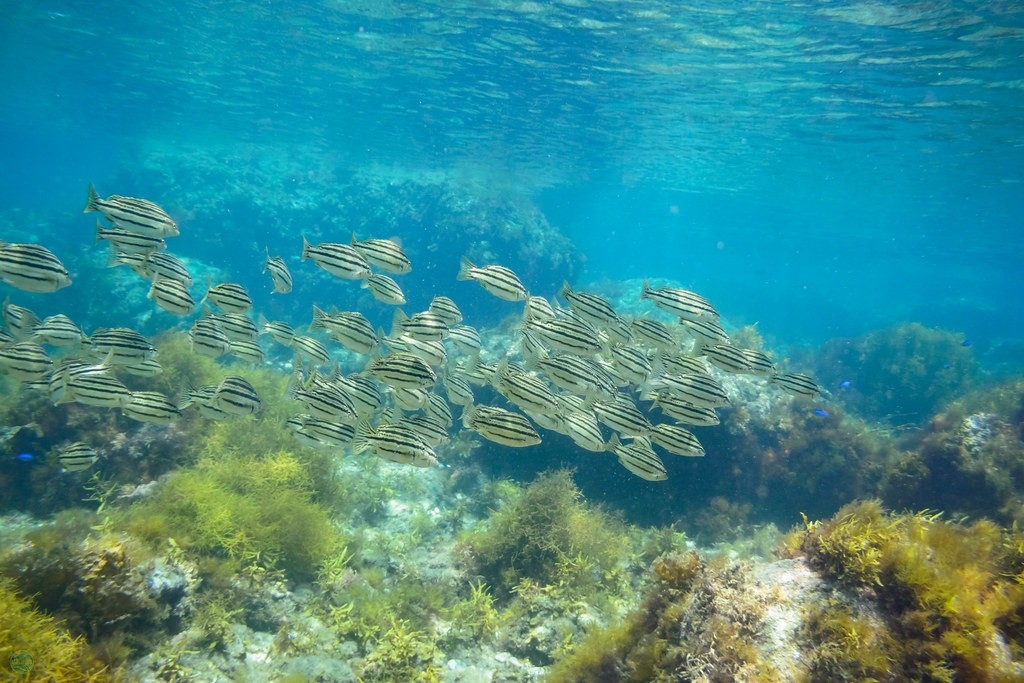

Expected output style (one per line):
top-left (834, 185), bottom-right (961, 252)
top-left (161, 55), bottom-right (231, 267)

top-left (0, 183), bottom-right (821, 482)
